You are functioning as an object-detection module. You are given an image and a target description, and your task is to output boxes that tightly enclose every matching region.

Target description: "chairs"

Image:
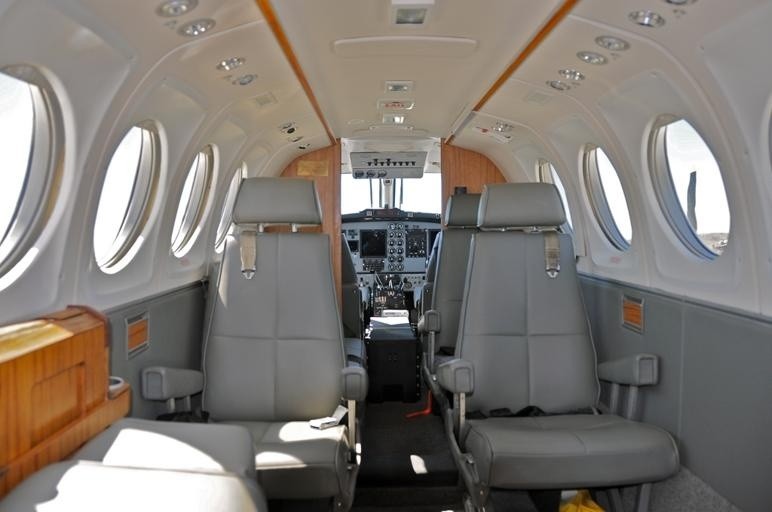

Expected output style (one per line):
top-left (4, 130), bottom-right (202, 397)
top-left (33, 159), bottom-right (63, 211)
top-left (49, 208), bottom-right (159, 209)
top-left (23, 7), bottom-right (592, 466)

top-left (408, 180), bottom-right (683, 509)
top-left (137, 170), bottom-right (375, 512)
